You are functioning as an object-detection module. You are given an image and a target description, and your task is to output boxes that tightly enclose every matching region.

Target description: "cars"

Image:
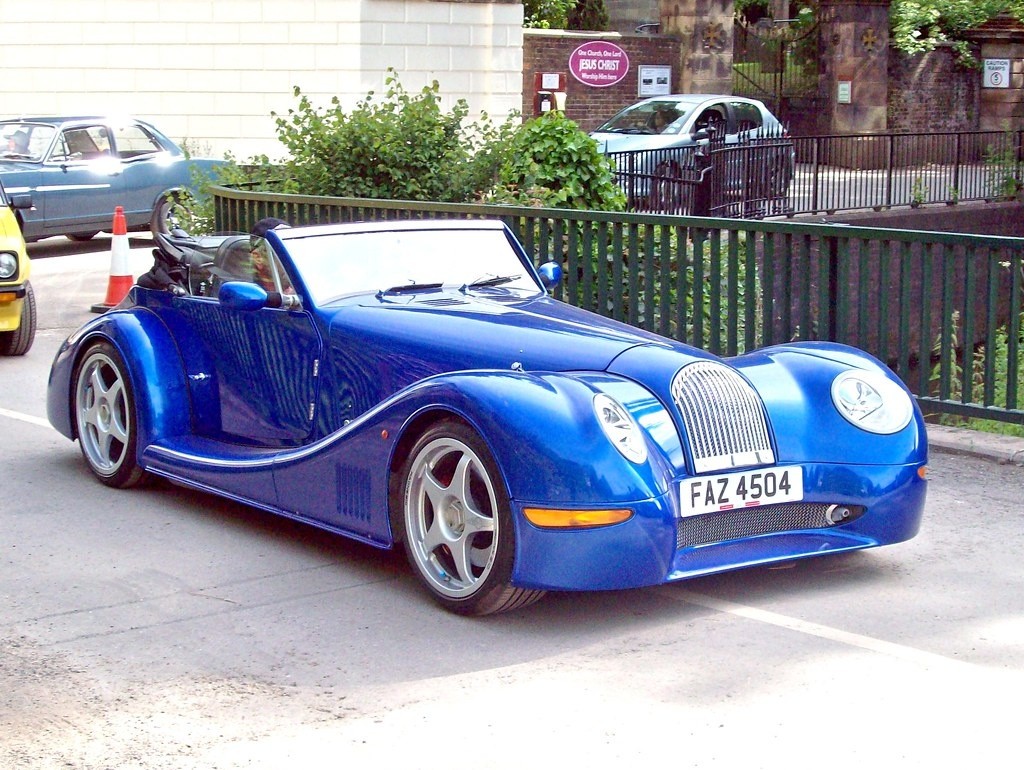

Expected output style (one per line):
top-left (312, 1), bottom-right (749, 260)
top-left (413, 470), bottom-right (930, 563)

top-left (0, 112), bottom-right (231, 246)
top-left (0, 178), bottom-right (33, 358)
top-left (586, 94), bottom-right (797, 210)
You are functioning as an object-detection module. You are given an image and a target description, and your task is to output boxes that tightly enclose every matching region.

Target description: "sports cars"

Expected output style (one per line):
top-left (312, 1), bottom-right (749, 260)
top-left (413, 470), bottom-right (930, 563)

top-left (46, 221), bottom-right (930, 616)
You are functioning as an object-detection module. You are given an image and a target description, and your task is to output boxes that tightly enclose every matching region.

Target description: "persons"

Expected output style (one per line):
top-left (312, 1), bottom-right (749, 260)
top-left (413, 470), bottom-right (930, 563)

top-left (630, 109), bottom-right (669, 134)
top-left (249, 218), bottom-right (296, 309)
top-left (3, 130), bottom-right (31, 153)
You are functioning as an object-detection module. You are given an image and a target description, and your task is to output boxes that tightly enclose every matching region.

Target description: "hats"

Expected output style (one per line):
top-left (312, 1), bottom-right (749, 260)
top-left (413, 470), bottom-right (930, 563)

top-left (249, 217), bottom-right (290, 252)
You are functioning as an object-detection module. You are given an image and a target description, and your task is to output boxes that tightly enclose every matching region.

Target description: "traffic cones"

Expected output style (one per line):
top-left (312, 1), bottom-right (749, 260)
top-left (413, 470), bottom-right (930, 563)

top-left (90, 206), bottom-right (136, 313)
